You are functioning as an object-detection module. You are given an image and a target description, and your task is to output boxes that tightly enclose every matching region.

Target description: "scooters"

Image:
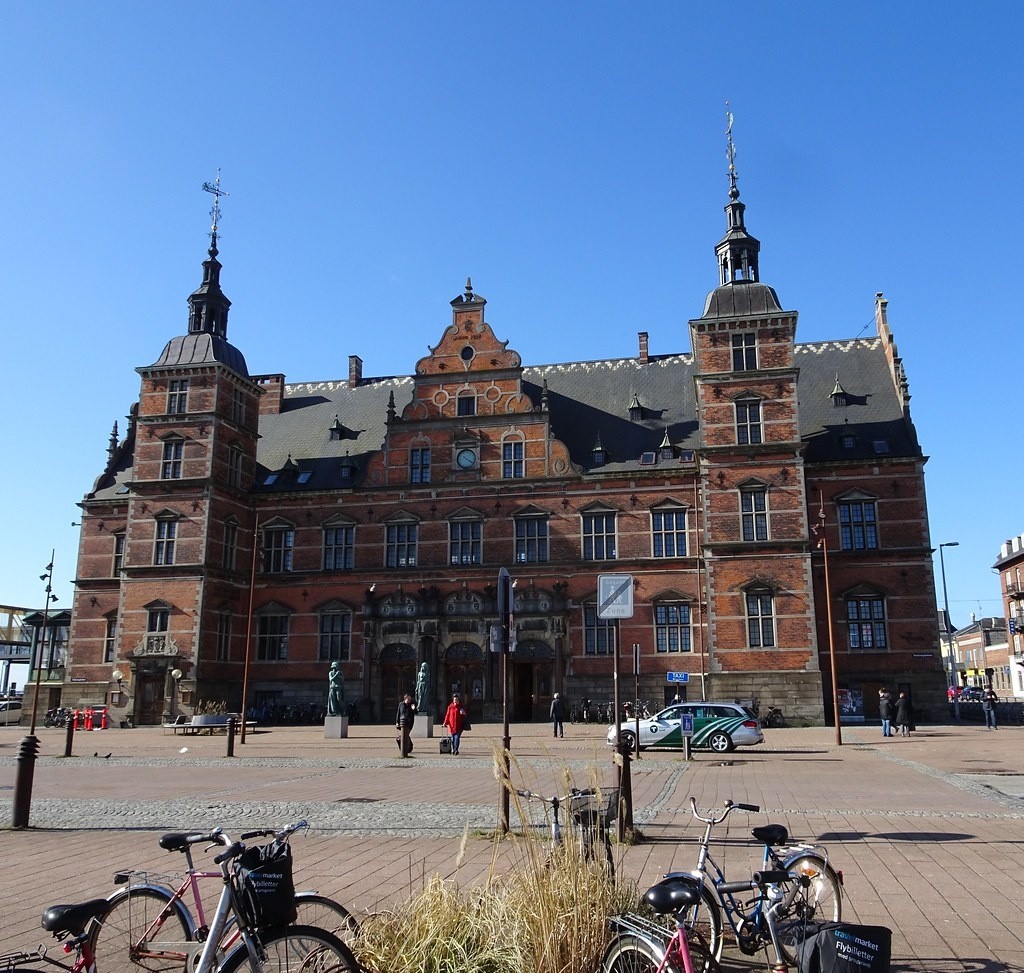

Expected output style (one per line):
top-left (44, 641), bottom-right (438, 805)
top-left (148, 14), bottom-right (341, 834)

top-left (750, 697), bottom-right (785, 728)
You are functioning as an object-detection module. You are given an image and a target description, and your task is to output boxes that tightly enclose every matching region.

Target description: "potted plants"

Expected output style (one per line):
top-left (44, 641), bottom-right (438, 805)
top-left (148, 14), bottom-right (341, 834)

top-left (190, 697), bottom-right (230, 726)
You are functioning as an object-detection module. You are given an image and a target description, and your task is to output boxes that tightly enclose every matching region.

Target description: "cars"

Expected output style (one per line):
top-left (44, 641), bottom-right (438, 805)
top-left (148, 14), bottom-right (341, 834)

top-left (960, 687), bottom-right (985, 701)
top-left (947, 685), bottom-right (965, 701)
top-left (606, 699), bottom-right (764, 754)
top-left (0, 691), bottom-right (24, 726)
top-left (837, 688), bottom-right (863, 715)
top-left (87, 704), bottom-right (105, 729)
top-left (44, 705), bottom-right (84, 730)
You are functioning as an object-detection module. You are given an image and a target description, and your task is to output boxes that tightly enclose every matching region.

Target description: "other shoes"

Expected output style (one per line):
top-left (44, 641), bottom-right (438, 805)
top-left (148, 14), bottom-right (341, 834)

top-left (560, 734), bottom-right (563, 738)
top-left (454, 750), bottom-right (459, 756)
top-left (554, 734), bottom-right (557, 737)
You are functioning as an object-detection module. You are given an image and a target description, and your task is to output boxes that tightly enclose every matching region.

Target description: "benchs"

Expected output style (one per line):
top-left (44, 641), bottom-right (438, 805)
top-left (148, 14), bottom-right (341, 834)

top-left (226, 713), bottom-right (257, 734)
top-left (161, 715), bottom-right (186, 735)
top-left (182, 715), bottom-right (229, 735)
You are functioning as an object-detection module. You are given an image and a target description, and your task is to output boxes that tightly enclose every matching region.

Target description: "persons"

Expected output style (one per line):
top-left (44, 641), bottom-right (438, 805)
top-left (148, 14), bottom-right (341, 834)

top-left (396, 694), bottom-right (418, 759)
top-left (550, 693), bottom-right (567, 738)
top-left (443, 694), bottom-right (467, 756)
top-left (880, 693), bottom-right (894, 737)
top-left (894, 692), bottom-right (910, 737)
top-left (980, 685), bottom-right (999, 730)
top-left (415, 662), bottom-right (432, 717)
top-left (328, 661), bottom-right (343, 718)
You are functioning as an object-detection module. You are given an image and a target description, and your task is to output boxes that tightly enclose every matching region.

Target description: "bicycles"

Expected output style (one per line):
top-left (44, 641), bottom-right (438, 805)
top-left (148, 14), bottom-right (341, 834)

top-left (87, 818), bottom-right (365, 973)
top-left (515, 785), bottom-right (620, 885)
top-left (653, 796), bottom-right (845, 967)
top-left (594, 703), bottom-right (607, 725)
top-left (577, 697), bottom-right (592, 725)
top-left (569, 703), bottom-right (581, 724)
top-left (0, 821), bottom-right (360, 973)
top-left (605, 699), bottom-right (668, 726)
top-left (597, 870), bottom-right (893, 973)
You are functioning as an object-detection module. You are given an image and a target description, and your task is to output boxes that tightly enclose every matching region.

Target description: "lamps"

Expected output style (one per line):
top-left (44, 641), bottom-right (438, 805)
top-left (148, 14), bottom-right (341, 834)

top-left (112, 671), bottom-right (124, 692)
top-left (172, 668), bottom-right (184, 690)
top-left (71, 521), bottom-right (82, 527)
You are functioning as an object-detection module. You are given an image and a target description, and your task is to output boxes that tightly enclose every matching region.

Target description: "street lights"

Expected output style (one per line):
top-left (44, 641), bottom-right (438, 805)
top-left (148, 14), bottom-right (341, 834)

top-left (240, 513), bottom-right (260, 744)
top-left (30, 547), bottom-right (60, 734)
top-left (940, 542), bottom-right (961, 720)
top-left (812, 489), bottom-right (842, 744)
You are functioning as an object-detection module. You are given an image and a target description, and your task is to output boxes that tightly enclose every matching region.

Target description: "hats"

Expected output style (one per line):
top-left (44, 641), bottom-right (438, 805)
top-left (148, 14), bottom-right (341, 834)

top-left (900, 692), bottom-right (905, 698)
top-left (554, 693), bottom-right (559, 698)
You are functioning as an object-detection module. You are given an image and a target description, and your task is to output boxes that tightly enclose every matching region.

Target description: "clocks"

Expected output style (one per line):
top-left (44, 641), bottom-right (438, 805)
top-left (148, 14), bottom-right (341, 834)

top-left (457, 449), bottom-right (476, 468)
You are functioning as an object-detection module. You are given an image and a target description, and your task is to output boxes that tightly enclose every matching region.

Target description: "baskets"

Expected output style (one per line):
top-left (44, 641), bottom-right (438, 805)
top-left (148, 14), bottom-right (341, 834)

top-left (572, 787), bottom-right (619, 823)
top-left (232, 841), bottom-right (297, 929)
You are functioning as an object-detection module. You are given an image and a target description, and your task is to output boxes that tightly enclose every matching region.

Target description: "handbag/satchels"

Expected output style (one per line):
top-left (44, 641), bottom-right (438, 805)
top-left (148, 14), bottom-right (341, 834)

top-left (791, 921), bottom-right (893, 973)
top-left (462, 715), bottom-right (471, 730)
top-left (910, 723), bottom-right (915, 731)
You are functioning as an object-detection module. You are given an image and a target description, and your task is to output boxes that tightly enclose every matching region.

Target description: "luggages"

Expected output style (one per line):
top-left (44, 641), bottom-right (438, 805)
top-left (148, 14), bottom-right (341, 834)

top-left (396, 735), bottom-right (413, 753)
top-left (439, 726), bottom-right (451, 754)
